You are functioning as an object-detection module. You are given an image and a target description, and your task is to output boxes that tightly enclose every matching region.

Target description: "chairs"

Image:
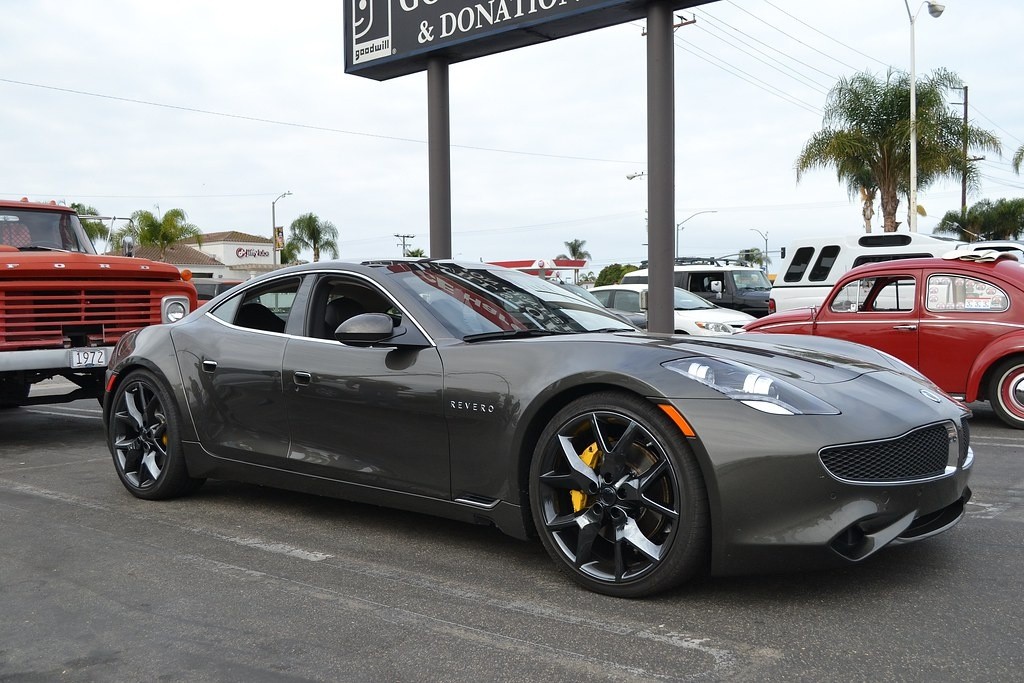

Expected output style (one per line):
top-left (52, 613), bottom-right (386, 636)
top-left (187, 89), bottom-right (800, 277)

top-left (235, 302), bottom-right (286, 332)
top-left (2, 223), bottom-right (32, 248)
top-left (325, 297), bottom-right (369, 340)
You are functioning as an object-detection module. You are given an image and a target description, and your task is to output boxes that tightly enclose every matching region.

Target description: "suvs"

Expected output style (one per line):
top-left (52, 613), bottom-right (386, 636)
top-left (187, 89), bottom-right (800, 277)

top-left (768, 233), bottom-right (1024, 313)
top-left (0, 196), bottom-right (200, 405)
top-left (621, 257), bottom-right (773, 319)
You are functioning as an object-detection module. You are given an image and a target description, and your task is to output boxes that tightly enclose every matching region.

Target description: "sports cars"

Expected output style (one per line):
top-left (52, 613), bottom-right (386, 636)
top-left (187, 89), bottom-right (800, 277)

top-left (99, 259), bottom-right (981, 600)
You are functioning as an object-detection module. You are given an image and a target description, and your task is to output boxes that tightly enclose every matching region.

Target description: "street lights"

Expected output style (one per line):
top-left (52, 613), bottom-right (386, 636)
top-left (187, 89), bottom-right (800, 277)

top-left (272, 192), bottom-right (293, 270)
top-left (904, 0), bottom-right (946, 233)
top-left (676, 210), bottom-right (716, 263)
top-left (749, 229), bottom-right (769, 280)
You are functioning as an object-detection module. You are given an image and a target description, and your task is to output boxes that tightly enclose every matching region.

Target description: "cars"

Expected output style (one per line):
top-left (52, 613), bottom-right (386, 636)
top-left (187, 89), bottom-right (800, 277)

top-left (729, 249), bottom-right (1024, 427)
top-left (587, 283), bottom-right (758, 340)
top-left (188, 279), bottom-right (245, 312)
